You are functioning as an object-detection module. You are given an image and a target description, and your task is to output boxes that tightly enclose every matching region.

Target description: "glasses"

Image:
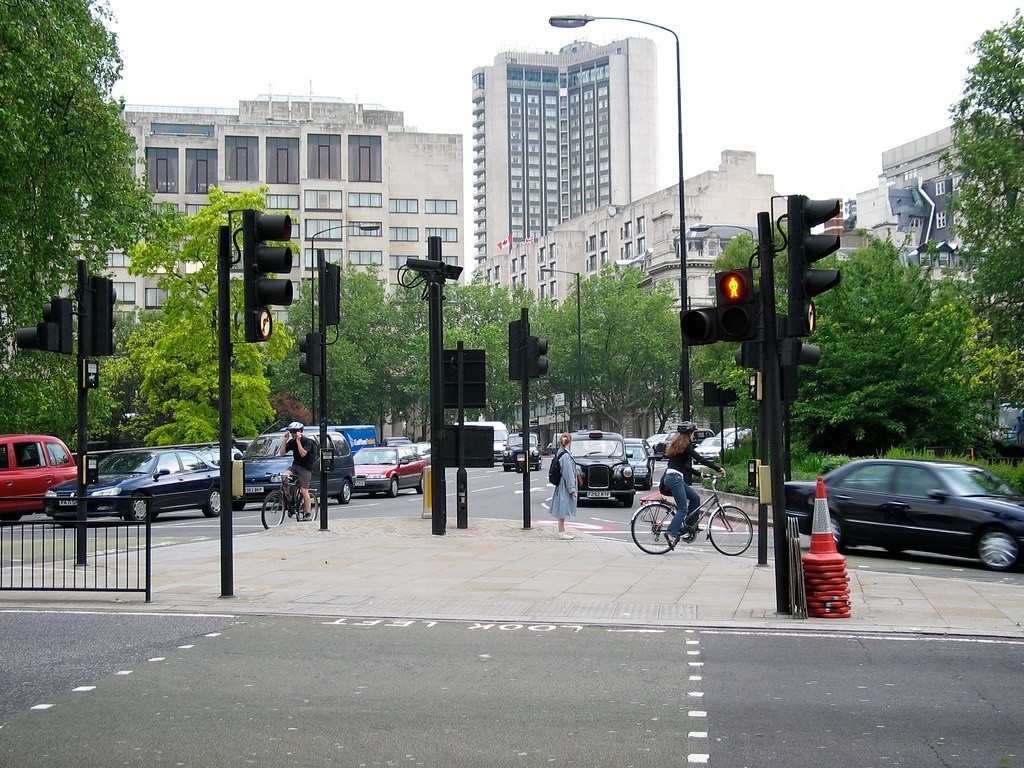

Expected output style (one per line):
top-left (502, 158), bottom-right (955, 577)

top-left (290, 430), bottom-right (298, 433)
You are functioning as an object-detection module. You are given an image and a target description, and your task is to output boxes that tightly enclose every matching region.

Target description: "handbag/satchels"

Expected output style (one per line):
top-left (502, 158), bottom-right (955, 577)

top-left (659, 476), bottom-right (673, 496)
top-left (577, 476), bottom-right (585, 489)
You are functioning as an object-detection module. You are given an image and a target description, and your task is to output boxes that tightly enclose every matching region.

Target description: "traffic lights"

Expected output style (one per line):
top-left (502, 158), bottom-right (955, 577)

top-left (733, 340), bottom-right (757, 369)
top-left (298, 333), bottom-right (322, 379)
top-left (527, 334), bottom-right (548, 378)
top-left (91, 277), bottom-right (119, 357)
top-left (679, 306), bottom-right (720, 346)
top-left (16, 296), bottom-right (74, 355)
top-left (241, 209), bottom-right (294, 341)
top-left (776, 314), bottom-right (821, 403)
top-left (786, 193), bottom-right (843, 336)
top-left (721, 388), bottom-right (737, 406)
top-left (712, 268), bottom-right (756, 341)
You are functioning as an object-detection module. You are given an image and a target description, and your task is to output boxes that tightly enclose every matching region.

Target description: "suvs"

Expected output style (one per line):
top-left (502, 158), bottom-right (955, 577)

top-left (231, 431), bottom-right (355, 510)
top-left (0, 434), bottom-right (78, 520)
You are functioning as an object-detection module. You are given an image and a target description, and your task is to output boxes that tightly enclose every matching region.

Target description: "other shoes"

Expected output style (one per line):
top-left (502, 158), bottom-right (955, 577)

top-left (303, 514), bottom-right (313, 521)
top-left (664, 533), bottom-right (675, 551)
top-left (557, 531), bottom-right (575, 540)
top-left (689, 525), bottom-right (703, 531)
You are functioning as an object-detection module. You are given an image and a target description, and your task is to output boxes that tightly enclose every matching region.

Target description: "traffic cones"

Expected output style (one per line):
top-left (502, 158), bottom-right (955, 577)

top-left (800, 475), bottom-right (852, 618)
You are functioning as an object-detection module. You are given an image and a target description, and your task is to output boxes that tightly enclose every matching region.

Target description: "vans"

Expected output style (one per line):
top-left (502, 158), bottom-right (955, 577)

top-left (280, 425), bottom-right (378, 459)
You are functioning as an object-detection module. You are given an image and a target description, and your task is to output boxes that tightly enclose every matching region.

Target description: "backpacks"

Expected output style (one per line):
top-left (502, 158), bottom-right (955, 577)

top-left (549, 451), bottom-right (568, 484)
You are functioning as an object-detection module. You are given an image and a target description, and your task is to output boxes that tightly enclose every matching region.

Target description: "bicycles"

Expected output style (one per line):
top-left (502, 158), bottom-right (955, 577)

top-left (629, 471), bottom-right (754, 556)
top-left (260, 472), bottom-right (319, 530)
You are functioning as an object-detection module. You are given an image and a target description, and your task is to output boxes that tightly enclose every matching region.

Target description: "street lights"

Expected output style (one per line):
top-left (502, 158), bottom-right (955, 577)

top-left (311, 223), bottom-right (381, 530)
top-left (690, 223), bottom-right (756, 492)
top-left (547, 12), bottom-right (692, 490)
top-left (540, 268), bottom-right (582, 431)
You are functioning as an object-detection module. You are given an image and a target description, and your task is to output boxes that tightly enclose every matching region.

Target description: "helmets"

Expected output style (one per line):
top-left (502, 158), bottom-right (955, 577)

top-left (677, 421), bottom-right (697, 433)
top-left (286, 422), bottom-right (304, 430)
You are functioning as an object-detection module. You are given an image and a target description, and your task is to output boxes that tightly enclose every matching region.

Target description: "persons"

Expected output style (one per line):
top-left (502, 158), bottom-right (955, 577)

top-left (663, 421), bottom-right (726, 551)
top-left (549, 432), bottom-right (578, 539)
top-left (279, 422), bottom-right (317, 522)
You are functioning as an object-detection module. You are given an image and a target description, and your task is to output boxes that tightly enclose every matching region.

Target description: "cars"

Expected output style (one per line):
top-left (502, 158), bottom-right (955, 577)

top-left (41, 438), bottom-right (257, 524)
top-left (784, 458), bottom-right (1024, 572)
top-left (352, 420), bottom-right (752, 500)
top-left (974, 402), bottom-right (1024, 455)
